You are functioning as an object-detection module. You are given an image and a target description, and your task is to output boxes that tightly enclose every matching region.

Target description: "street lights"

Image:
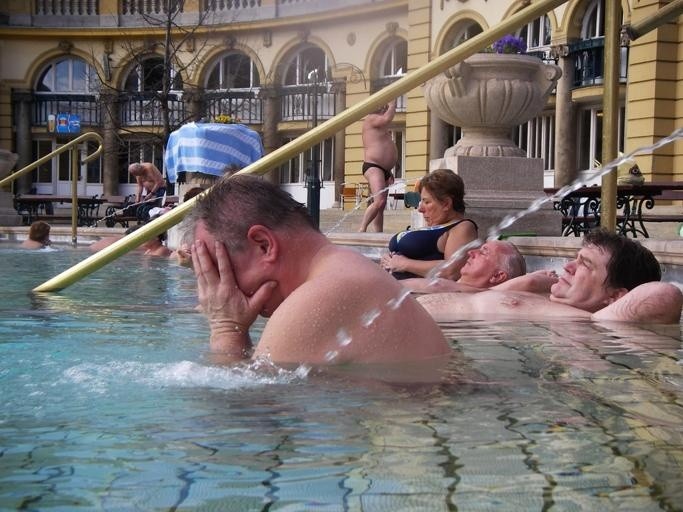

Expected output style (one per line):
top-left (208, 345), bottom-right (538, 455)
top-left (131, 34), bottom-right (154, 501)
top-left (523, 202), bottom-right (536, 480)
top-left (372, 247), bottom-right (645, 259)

top-left (306, 66), bottom-right (320, 232)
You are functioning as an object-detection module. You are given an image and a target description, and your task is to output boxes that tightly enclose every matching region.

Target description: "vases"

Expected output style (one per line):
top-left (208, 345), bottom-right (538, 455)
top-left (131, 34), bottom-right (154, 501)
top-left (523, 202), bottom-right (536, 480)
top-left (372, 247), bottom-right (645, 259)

top-left (422, 53), bottom-right (563, 158)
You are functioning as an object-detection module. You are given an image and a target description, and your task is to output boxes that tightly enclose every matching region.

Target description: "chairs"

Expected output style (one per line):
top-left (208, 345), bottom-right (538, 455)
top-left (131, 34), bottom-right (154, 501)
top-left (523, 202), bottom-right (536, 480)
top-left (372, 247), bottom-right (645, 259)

top-left (105, 193), bottom-right (156, 228)
top-left (340, 182), bottom-right (362, 210)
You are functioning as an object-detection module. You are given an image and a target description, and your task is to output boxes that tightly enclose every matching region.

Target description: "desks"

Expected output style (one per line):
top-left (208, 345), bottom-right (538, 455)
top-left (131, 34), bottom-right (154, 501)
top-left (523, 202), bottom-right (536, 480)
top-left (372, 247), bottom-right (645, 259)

top-left (543, 182), bottom-right (683, 239)
top-left (14, 194), bottom-right (108, 227)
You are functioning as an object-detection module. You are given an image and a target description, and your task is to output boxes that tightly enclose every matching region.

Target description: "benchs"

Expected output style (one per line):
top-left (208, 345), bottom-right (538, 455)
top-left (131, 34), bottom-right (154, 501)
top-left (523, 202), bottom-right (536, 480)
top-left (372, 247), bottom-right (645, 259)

top-left (563, 214), bottom-right (683, 238)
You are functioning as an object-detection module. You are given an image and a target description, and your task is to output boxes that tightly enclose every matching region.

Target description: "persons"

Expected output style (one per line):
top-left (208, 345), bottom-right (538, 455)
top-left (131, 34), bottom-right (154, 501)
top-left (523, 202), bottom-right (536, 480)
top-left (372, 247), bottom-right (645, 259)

top-left (358, 97), bottom-right (398, 232)
top-left (190, 162), bottom-right (454, 386)
top-left (129, 163), bottom-right (167, 224)
top-left (141, 214), bottom-right (170, 257)
top-left (89, 224), bottom-right (141, 252)
top-left (397, 240), bottom-right (527, 295)
top-left (20, 221), bottom-right (52, 249)
top-left (380, 169), bottom-right (478, 280)
top-left (415, 227), bottom-right (683, 326)
top-left (177, 187), bottom-right (206, 272)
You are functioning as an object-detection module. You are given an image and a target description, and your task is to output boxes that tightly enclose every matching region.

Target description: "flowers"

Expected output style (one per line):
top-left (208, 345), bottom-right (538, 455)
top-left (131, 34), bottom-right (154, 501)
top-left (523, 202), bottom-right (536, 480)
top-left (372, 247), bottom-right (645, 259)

top-left (493, 33), bottom-right (527, 55)
